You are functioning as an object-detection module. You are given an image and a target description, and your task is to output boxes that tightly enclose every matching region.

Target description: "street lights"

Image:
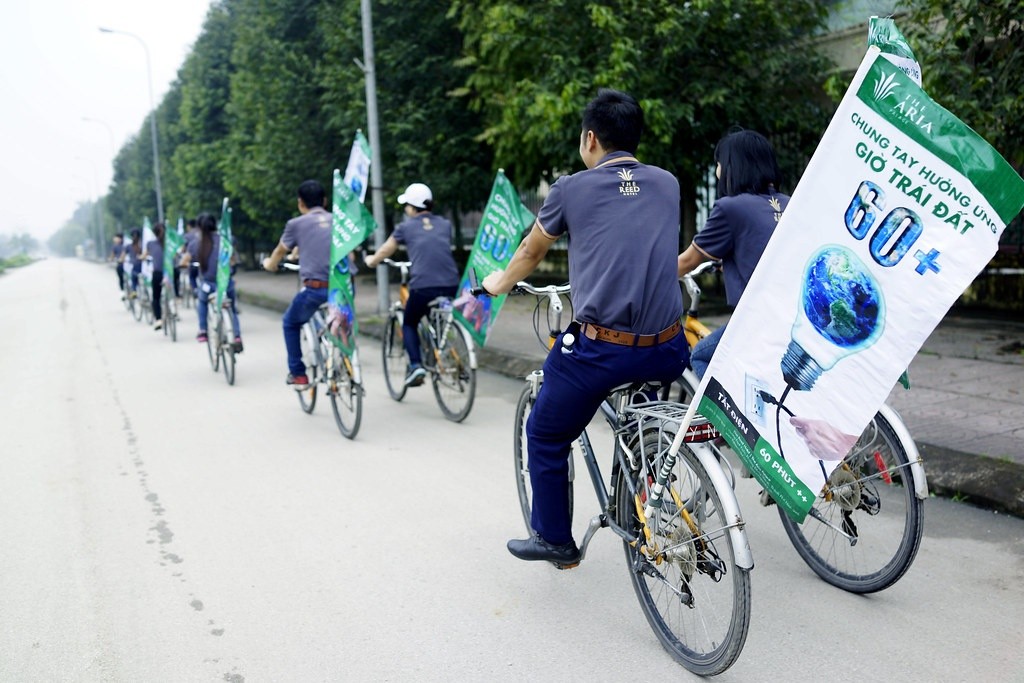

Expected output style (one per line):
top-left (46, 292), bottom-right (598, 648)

top-left (97, 25), bottom-right (165, 226)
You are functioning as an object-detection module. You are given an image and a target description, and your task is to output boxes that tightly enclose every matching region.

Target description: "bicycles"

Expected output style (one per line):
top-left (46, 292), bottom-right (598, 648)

top-left (466, 276), bottom-right (755, 677)
top-left (670, 257), bottom-right (934, 594)
top-left (367, 256), bottom-right (480, 425)
top-left (257, 258), bottom-right (371, 442)
top-left (107, 253), bottom-right (243, 389)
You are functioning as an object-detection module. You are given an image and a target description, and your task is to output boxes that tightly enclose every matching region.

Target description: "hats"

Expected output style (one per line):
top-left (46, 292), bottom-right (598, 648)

top-left (398, 183), bottom-right (432, 208)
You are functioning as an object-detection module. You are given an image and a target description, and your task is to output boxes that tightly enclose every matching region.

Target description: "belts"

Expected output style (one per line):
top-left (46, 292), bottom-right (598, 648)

top-left (580, 319), bottom-right (682, 347)
top-left (304, 279), bottom-right (328, 288)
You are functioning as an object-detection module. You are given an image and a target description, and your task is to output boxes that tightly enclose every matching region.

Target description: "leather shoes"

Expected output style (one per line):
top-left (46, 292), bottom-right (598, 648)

top-left (507, 535), bottom-right (580, 563)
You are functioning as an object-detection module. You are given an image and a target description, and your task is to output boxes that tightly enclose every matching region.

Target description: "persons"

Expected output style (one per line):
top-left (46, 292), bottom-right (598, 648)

top-left (482, 92), bottom-right (690, 563)
top-left (263, 180), bottom-right (332, 389)
top-left (790, 415), bottom-right (858, 461)
top-left (109, 224), bottom-right (165, 330)
top-left (174, 214), bottom-right (242, 352)
top-left (365, 184), bottom-right (458, 386)
top-left (676, 132), bottom-right (790, 448)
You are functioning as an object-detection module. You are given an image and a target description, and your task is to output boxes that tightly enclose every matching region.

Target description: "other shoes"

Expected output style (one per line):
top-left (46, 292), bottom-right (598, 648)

top-left (153, 320), bottom-right (162, 331)
top-left (197, 333), bottom-right (208, 342)
top-left (287, 376), bottom-right (309, 390)
top-left (405, 364), bottom-right (425, 385)
top-left (128, 291), bottom-right (138, 302)
top-left (235, 338), bottom-right (243, 352)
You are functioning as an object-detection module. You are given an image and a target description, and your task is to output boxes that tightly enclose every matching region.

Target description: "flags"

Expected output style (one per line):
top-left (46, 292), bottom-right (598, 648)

top-left (700, 55), bottom-right (1024, 525)
top-left (869, 17), bottom-right (923, 389)
top-left (123, 216), bottom-right (185, 299)
top-left (452, 174), bottom-right (537, 348)
top-left (216, 208), bottom-right (233, 311)
top-left (329, 132), bottom-right (378, 356)
